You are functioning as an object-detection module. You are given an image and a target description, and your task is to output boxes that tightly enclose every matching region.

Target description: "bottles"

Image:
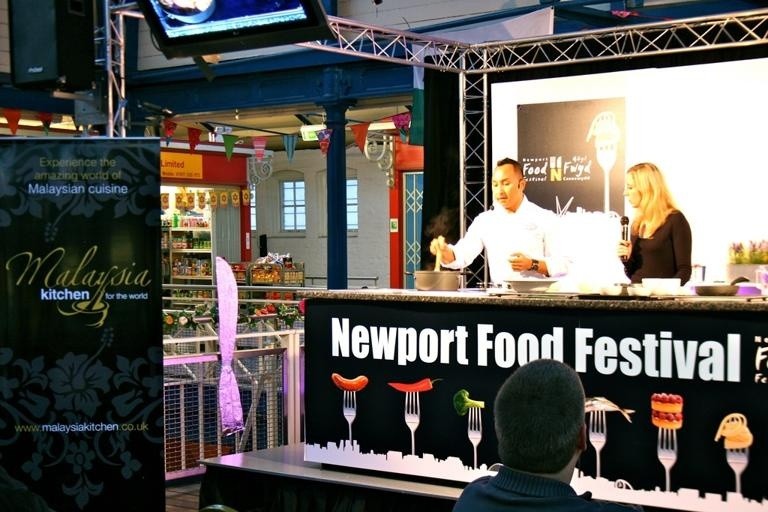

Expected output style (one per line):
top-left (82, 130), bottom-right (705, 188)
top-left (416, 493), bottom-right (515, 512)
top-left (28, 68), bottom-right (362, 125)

top-left (172, 212), bottom-right (210, 249)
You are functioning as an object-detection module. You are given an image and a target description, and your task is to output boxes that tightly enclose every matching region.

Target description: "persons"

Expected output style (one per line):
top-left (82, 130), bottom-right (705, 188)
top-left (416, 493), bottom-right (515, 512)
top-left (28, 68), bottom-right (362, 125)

top-left (429, 157), bottom-right (569, 288)
top-left (452, 359), bottom-right (643, 512)
top-left (616, 162), bottom-right (692, 286)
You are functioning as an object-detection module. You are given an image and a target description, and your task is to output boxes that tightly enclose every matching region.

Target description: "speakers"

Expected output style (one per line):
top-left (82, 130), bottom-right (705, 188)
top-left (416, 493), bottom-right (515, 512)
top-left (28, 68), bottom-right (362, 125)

top-left (8, 0), bottom-right (96, 90)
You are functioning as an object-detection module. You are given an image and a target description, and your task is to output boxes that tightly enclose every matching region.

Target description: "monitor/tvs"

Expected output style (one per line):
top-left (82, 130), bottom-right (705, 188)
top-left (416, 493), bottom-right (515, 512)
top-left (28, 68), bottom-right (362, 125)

top-left (136, 0), bottom-right (337, 60)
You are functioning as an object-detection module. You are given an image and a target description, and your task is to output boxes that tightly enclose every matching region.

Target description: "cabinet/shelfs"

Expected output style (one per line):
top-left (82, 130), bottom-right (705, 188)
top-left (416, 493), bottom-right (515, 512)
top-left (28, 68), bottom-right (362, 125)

top-left (161, 185), bottom-right (305, 310)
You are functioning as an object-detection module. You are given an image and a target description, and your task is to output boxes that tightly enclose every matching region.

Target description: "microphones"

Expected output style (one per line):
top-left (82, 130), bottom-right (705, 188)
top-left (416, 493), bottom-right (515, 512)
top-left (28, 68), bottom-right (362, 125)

top-left (620, 216), bottom-right (629, 265)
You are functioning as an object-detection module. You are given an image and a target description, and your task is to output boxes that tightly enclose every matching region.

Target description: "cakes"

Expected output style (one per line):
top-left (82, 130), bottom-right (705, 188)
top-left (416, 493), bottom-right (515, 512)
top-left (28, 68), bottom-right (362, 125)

top-left (650, 392), bottom-right (684, 430)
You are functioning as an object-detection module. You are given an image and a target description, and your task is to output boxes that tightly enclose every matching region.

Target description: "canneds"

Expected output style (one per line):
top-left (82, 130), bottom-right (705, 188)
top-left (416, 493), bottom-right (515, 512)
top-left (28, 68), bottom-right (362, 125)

top-left (172, 258), bottom-right (195, 275)
top-left (192, 291), bottom-right (209, 298)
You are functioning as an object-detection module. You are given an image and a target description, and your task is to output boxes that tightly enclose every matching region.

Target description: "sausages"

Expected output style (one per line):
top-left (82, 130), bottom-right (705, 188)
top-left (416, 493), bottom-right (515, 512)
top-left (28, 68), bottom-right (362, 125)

top-left (332, 373), bottom-right (369, 391)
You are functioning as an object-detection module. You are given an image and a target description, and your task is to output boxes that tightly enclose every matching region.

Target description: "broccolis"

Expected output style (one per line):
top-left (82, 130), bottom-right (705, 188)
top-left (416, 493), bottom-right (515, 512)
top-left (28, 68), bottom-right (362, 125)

top-left (453, 388), bottom-right (485, 416)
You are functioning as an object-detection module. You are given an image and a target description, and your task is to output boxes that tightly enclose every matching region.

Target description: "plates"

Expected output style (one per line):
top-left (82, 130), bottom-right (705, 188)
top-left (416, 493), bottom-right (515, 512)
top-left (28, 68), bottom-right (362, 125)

top-left (504, 279), bottom-right (559, 292)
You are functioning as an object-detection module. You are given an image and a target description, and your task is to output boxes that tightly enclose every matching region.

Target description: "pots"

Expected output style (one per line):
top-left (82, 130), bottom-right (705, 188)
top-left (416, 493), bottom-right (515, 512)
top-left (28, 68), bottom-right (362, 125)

top-left (694, 277), bottom-right (749, 296)
top-left (403, 271), bottom-right (473, 291)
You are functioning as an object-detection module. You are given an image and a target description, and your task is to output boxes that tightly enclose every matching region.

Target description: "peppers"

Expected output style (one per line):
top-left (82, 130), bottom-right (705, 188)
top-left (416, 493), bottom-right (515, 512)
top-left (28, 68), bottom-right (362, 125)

top-left (387, 377), bottom-right (444, 392)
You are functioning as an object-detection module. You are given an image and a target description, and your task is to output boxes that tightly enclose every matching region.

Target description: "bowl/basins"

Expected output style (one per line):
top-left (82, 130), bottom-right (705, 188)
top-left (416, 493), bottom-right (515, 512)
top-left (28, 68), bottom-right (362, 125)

top-left (642, 277), bottom-right (681, 295)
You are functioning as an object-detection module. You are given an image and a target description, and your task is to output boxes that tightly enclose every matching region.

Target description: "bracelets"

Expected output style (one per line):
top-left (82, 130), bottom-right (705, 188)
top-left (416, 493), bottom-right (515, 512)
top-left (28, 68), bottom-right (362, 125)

top-left (528, 259), bottom-right (539, 273)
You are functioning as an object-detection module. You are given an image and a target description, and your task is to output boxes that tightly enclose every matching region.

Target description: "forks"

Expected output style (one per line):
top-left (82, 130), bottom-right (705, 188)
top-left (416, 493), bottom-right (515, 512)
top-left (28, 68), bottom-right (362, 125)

top-left (342, 390), bottom-right (357, 443)
top-left (468, 406), bottom-right (484, 468)
top-left (404, 390), bottom-right (421, 453)
top-left (588, 411), bottom-right (607, 478)
top-left (727, 447), bottom-right (750, 495)
top-left (657, 427), bottom-right (678, 493)
